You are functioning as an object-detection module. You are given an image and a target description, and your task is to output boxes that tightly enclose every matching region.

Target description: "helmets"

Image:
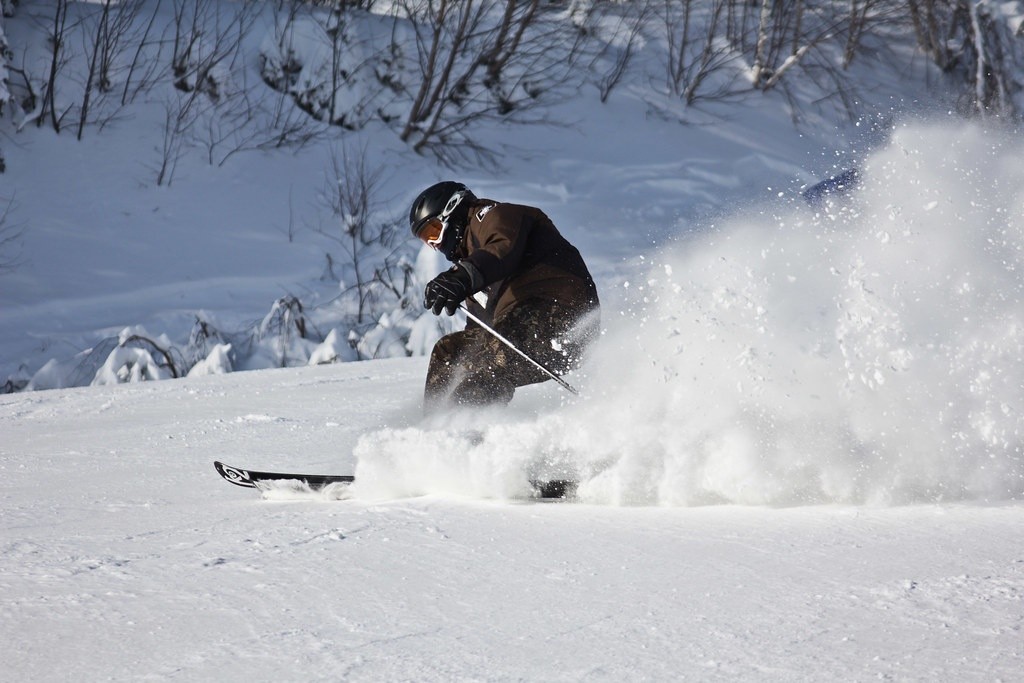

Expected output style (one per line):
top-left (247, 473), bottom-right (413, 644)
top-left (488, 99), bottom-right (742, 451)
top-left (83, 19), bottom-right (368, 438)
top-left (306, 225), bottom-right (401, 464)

top-left (410, 181), bottom-right (480, 263)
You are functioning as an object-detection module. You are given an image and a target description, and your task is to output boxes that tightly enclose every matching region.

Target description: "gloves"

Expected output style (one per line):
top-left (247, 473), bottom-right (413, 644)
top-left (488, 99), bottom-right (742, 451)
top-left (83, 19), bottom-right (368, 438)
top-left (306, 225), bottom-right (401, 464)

top-left (422, 262), bottom-right (472, 317)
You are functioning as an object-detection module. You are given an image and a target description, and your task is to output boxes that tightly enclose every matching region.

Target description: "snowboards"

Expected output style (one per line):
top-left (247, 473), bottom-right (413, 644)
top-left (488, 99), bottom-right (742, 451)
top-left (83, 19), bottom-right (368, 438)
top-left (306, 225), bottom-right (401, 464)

top-left (213, 460), bottom-right (604, 499)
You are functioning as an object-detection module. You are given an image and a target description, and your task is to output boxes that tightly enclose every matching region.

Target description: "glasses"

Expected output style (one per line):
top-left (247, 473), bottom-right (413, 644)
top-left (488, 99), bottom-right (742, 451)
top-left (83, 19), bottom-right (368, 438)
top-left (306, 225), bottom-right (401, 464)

top-left (415, 216), bottom-right (449, 252)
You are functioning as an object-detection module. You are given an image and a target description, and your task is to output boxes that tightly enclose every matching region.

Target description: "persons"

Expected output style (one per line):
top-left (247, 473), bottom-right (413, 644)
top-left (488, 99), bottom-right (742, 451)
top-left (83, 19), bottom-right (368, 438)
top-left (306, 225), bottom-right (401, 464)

top-left (410, 181), bottom-right (601, 411)
